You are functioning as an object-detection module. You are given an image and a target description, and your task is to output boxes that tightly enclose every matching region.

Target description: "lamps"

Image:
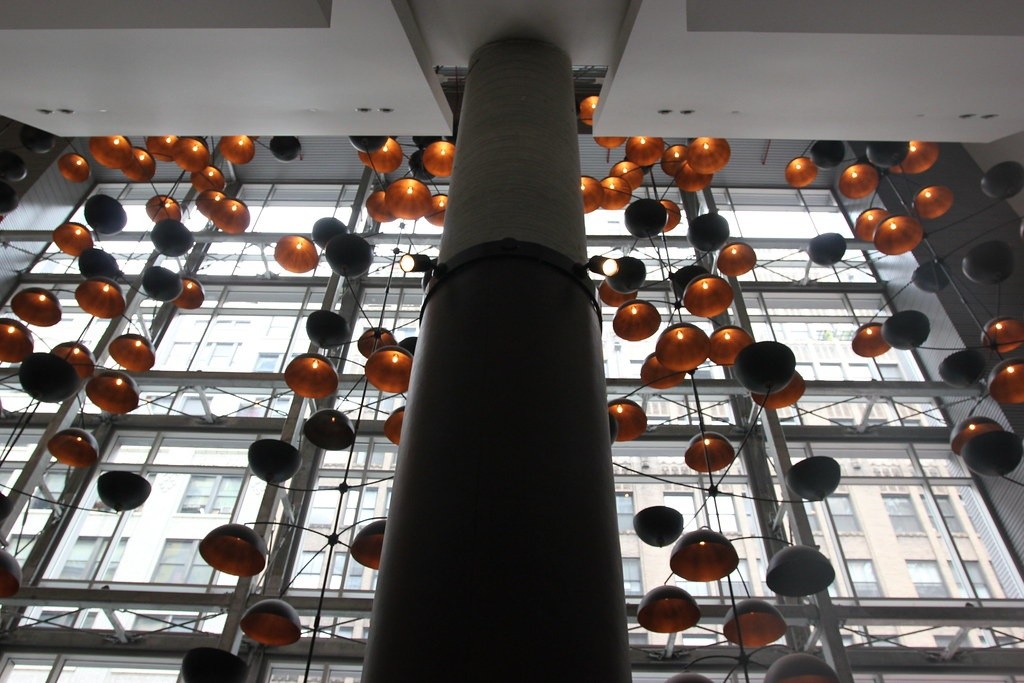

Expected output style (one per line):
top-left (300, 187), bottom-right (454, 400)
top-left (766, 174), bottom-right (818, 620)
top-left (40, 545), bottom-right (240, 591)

top-left (0, 94), bottom-right (1024, 683)
